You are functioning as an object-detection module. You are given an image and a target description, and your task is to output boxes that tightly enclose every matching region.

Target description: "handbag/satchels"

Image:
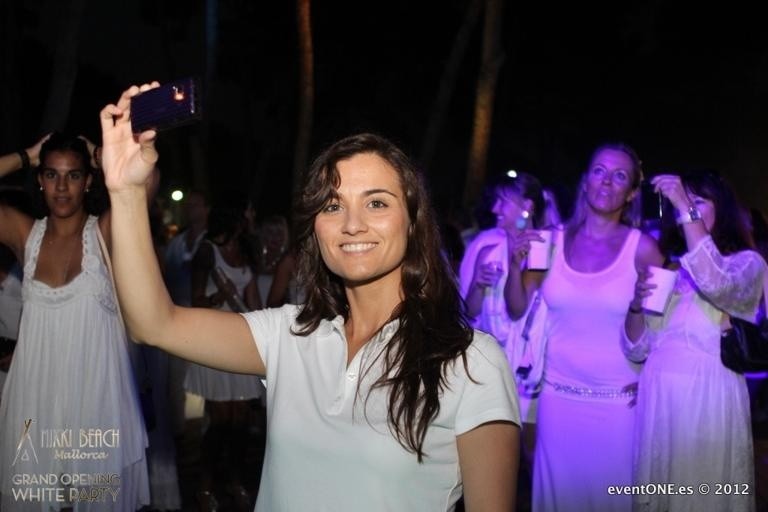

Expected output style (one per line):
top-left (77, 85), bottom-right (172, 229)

top-left (717, 312), bottom-right (768, 374)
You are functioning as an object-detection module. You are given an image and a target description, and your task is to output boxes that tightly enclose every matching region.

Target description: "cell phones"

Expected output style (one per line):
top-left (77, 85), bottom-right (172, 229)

top-left (641, 180), bottom-right (663, 220)
top-left (130, 76), bottom-right (202, 134)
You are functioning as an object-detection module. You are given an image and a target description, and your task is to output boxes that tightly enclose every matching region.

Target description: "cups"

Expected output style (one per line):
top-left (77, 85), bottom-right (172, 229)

top-left (639, 265), bottom-right (678, 314)
top-left (526, 229), bottom-right (552, 271)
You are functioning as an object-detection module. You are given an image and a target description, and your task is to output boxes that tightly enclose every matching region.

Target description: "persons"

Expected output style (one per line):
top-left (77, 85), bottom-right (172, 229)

top-left (100, 78), bottom-right (524, 512)
top-left (0, 127), bottom-right (768, 512)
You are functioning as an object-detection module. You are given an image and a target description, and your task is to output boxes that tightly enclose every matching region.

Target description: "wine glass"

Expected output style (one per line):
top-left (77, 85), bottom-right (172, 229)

top-left (484, 260), bottom-right (506, 317)
